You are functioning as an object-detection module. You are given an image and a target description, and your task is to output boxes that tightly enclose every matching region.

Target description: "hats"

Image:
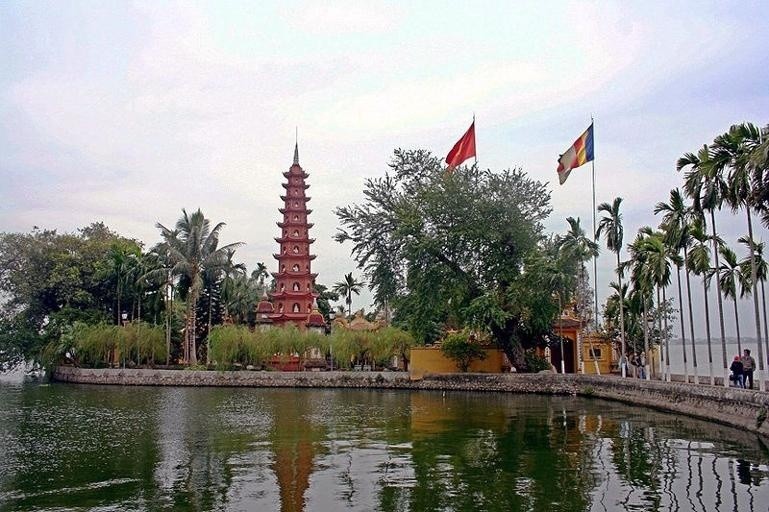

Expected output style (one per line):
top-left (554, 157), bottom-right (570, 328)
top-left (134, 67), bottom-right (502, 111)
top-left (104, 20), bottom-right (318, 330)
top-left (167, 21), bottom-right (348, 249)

top-left (734, 355), bottom-right (739, 361)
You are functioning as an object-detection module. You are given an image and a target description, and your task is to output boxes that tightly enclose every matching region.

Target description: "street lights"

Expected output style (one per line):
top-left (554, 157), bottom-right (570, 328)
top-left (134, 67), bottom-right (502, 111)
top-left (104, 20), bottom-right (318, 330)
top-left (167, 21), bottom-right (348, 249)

top-left (329, 308), bottom-right (336, 371)
top-left (122, 310), bottom-right (128, 369)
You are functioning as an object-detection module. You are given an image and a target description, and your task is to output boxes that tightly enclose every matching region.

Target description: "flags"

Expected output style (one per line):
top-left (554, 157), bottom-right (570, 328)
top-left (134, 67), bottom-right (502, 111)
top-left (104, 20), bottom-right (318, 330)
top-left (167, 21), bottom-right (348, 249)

top-left (445, 122), bottom-right (475, 172)
top-left (558, 124), bottom-right (594, 184)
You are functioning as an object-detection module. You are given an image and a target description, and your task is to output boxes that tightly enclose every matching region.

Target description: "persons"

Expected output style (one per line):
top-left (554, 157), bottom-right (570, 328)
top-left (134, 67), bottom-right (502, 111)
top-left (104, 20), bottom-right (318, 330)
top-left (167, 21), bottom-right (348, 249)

top-left (619, 352), bottom-right (629, 377)
top-left (630, 352), bottom-right (639, 378)
top-left (741, 349), bottom-right (756, 389)
top-left (730, 356), bottom-right (744, 389)
top-left (638, 352), bottom-right (646, 379)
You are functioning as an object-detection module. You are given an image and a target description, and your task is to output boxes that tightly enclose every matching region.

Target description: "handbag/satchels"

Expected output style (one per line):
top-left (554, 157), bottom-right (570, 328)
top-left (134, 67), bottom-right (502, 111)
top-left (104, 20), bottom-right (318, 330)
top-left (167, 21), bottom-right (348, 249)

top-left (729, 374), bottom-right (740, 381)
